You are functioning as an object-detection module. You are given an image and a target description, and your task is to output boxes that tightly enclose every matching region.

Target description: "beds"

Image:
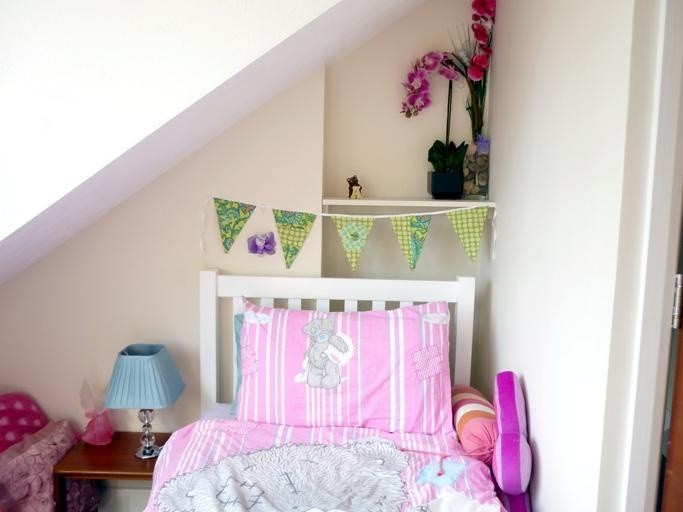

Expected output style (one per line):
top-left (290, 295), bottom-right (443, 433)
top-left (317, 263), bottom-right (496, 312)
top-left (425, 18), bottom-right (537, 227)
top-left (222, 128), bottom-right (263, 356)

top-left (134, 265), bottom-right (512, 511)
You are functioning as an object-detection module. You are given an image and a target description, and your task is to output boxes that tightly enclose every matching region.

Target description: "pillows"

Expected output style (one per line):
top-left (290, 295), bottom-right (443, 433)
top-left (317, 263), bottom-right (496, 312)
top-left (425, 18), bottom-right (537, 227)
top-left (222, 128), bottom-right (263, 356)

top-left (446, 379), bottom-right (502, 466)
top-left (0, 391), bottom-right (109, 511)
top-left (229, 312), bottom-right (242, 417)
top-left (233, 291), bottom-right (458, 441)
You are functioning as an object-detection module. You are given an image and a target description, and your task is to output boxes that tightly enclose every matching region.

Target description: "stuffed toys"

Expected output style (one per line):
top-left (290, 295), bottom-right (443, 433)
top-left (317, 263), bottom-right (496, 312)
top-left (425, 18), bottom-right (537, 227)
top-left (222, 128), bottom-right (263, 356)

top-left (346, 175), bottom-right (361, 199)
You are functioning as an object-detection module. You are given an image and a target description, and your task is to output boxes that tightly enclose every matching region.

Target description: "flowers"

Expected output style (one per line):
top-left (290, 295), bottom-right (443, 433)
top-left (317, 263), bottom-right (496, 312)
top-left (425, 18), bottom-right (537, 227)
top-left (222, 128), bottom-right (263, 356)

top-left (399, 0), bottom-right (493, 175)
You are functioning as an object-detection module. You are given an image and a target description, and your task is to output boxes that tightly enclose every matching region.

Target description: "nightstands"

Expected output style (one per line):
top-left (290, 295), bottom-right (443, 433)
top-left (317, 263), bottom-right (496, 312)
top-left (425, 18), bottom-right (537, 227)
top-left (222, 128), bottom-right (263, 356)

top-left (50, 430), bottom-right (170, 512)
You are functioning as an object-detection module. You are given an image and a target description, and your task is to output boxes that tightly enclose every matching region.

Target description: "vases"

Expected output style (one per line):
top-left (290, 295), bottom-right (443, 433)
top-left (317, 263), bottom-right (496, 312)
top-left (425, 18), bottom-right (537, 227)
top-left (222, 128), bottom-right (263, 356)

top-left (423, 164), bottom-right (461, 201)
top-left (459, 148), bottom-right (491, 201)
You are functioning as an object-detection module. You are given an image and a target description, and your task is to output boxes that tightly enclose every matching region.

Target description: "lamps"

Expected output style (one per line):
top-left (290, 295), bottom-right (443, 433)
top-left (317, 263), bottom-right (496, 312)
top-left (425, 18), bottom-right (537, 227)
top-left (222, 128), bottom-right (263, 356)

top-left (101, 342), bottom-right (188, 459)
